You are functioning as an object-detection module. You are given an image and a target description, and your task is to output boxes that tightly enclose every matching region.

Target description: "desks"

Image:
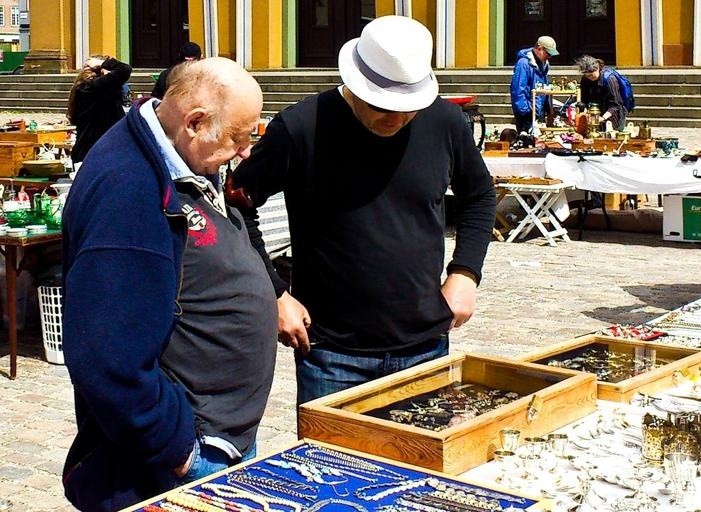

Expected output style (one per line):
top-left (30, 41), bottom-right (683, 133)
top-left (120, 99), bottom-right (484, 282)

top-left (118, 295), bottom-right (701, 511)
top-left (1, 226), bottom-right (61, 379)
top-left (546, 153), bottom-right (701, 240)
top-left (447, 153), bottom-right (548, 238)
top-left (123, 105), bottom-right (131, 115)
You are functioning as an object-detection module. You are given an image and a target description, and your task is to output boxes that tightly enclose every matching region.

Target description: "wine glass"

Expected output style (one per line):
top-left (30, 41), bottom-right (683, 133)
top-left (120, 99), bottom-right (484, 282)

top-left (662, 453), bottom-right (698, 506)
top-left (493, 430), bottom-right (568, 485)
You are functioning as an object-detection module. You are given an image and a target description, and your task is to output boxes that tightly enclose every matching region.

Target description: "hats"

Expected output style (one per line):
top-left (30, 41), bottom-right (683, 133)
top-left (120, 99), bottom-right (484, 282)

top-left (337, 15), bottom-right (440, 112)
top-left (177, 42), bottom-right (200, 60)
top-left (538, 35), bottom-right (560, 57)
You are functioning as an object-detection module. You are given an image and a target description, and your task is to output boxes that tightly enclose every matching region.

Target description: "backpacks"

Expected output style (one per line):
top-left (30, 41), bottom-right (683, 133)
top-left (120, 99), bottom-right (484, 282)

top-left (603, 67), bottom-right (634, 113)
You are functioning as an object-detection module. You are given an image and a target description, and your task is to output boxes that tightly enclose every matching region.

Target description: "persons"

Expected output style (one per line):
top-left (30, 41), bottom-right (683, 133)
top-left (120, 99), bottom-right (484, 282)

top-left (61, 57), bottom-right (276, 512)
top-left (509, 35), bottom-right (560, 209)
top-left (152, 41), bottom-right (201, 99)
top-left (578, 54), bottom-right (627, 209)
top-left (65, 55), bottom-right (133, 159)
top-left (231, 15), bottom-right (498, 445)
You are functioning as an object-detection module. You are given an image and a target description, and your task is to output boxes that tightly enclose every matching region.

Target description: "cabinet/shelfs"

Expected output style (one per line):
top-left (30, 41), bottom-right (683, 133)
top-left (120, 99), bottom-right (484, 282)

top-left (531, 88), bottom-right (582, 135)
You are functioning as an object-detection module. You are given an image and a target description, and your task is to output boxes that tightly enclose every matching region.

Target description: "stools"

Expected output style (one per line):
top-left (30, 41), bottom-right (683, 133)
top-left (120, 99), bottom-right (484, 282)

top-left (499, 182), bottom-right (575, 246)
top-left (450, 186), bottom-right (516, 242)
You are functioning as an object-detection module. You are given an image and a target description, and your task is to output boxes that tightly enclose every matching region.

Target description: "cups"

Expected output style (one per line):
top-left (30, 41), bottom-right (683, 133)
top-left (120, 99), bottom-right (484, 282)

top-left (258, 123), bottom-right (267, 136)
top-left (5, 193), bottom-right (63, 230)
top-left (616, 132), bottom-right (632, 140)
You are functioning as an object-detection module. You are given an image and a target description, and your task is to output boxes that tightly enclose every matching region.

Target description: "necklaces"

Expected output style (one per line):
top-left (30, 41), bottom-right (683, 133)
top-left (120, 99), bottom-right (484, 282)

top-left (131, 449), bottom-right (530, 512)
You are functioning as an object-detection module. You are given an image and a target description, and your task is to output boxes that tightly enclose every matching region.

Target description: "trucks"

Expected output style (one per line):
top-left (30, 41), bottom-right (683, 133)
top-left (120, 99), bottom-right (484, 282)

top-left (1, 51), bottom-right (30, 75)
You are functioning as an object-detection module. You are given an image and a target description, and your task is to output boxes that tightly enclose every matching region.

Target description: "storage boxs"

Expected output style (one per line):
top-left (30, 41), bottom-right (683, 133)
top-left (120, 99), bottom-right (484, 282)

top-left (297, 349), bottom-right (599, 475)
top-left (0, 132), bottom-right (68, 143)
top-left (661, 192), bottom-right (701, 244)
top-left (519, 333), bottom-right (701, 403)
top-left (594, 138), bottom-right (656, 154)
top-left (0, 140), bottom-right (34, 178)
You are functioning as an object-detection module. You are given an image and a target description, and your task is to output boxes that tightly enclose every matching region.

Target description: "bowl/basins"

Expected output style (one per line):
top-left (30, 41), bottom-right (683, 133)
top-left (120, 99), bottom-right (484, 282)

top-left (1, 225), bottom-right (48, 238)
top-left (22, 160), bottom-right (66, 177)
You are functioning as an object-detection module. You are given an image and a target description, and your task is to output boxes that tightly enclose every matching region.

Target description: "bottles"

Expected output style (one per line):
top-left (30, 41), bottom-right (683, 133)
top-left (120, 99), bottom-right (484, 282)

top-left (589, 103), bottom-right (602, 125)
top-left (30, 120), bottom-right (37, 130)
top-left (640, 120), bottom-right (651, 139)
